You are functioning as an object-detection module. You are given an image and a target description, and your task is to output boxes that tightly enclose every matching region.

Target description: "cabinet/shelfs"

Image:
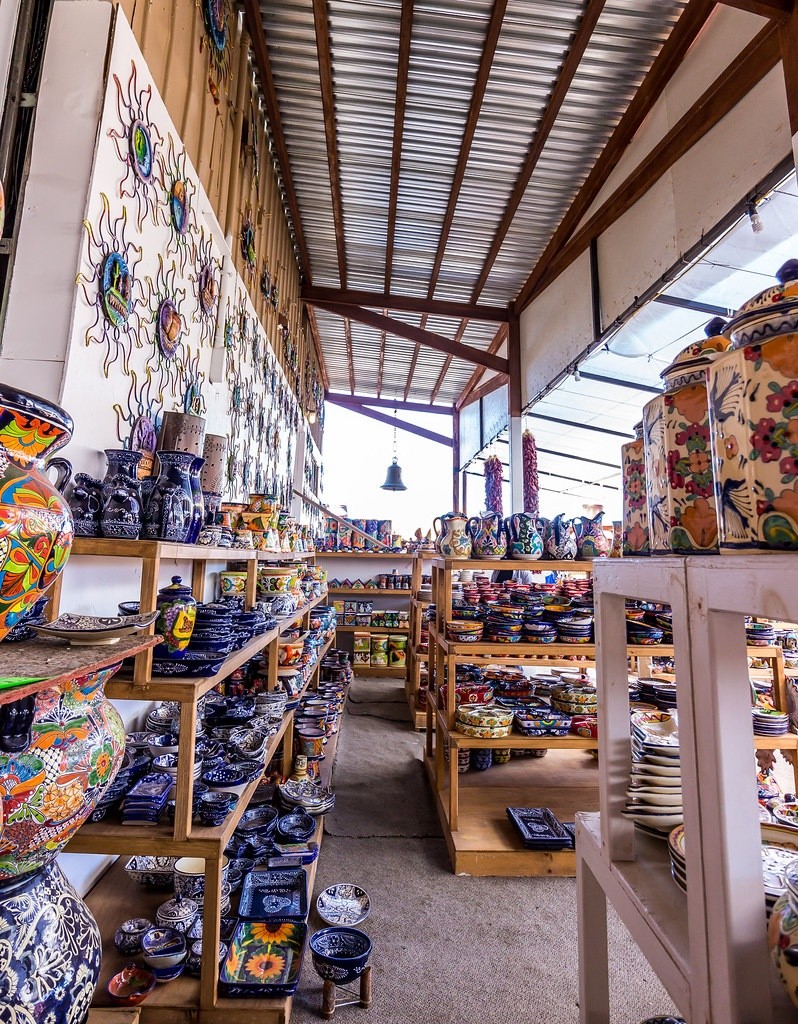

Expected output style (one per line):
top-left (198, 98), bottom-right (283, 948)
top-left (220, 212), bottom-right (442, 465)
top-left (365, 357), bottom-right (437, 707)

top-left (313, 552), bottom-right (419, 679)
top-left (405, 551), bottom-right (798, 1024)
top-left (42, 537), bottom-right (352, 1024)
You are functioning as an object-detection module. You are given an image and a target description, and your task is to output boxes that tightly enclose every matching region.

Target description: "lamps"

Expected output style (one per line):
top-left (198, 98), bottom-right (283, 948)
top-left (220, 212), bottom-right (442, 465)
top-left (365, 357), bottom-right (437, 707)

top-left (379, 398), bottom-right (408, 493)
top-left (306, 406), bottom-right (316, 424)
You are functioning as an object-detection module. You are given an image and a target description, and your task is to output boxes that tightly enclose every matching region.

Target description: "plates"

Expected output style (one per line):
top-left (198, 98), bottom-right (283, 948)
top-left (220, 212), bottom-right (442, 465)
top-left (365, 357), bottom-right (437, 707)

top-left (752, 708), bottom-right (789, 737)
top-left (193, 882), bottom-right (232, 917)
top-left (633, 825), bottom-right (682, 840)
top-left (620, 678), bottom-right (683, 826)
top-left (667, 826), bottom-right (798, 931)
top-left (25, 611), bottom-right (163, 646)
top-left (506, 807), bottom-right (575, 849)
top-left (745, 622), bottom-right (775, 644)
top-left (122, 772), bottom-right (172, 825)
top-left (317, 883), bottom-right (372, 926)
top-left (624, 597), bottom-right (674, 644)
top-left (447, 570), bottom-right (595, 643)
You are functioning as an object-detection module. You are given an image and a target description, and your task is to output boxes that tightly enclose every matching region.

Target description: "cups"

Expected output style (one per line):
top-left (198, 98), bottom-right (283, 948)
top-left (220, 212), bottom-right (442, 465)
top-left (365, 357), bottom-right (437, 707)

top-left (204, 526), bottom-right (220, 545)
top-left (252, 530), bottom-right (270, 550)
top-left (215, 512), bottom-right (231, 529)
top-left (231, 529), bottom-right (254, 550)
top-left (156, 892), bottom-right (198, 931)
top-left (196, 531), bottom-right (214, 545)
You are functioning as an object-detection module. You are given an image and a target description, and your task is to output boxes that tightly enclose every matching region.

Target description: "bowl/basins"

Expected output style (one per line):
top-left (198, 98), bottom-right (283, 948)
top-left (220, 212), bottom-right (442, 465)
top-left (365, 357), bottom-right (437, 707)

top-left (144, 928), bottom-right (187, 983)
top-left (309, 927), bottom-right (372, 985)
top-left (193, 940), bottom-right (229, 972)
top-left (106, 962), bottom-right (157, 1007)
top-left (222, 493), bottom-right (314, 551)
top-left (229, 858), bottom-right (255, 874)
top-left (174, 854), bottom-right (229, 898)
top-left (123, 690), bottom-right (300, 758)
top-left (186, 915), bottom-right (237, 942)
top-left (115, 918), bottom-right (154, 951)
top-left (193, 759), bottom-right (336, 841)
top-left (267, 842), bottom-right (319, 870)
top-left (263, 637), bottom-right (304, 698)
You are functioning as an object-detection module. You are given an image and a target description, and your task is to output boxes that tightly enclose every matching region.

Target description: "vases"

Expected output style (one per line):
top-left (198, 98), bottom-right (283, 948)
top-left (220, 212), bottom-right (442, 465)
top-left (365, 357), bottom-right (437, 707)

top-left (98, 448), bottom-right (207, 545)
top-left (434, 505), bottom-right (609, 561)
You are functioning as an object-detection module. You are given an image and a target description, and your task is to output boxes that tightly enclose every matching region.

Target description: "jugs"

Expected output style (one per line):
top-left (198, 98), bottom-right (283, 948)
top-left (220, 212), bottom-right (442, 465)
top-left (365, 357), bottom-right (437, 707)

top-left (433, 510), bottom-right (610, 560)
